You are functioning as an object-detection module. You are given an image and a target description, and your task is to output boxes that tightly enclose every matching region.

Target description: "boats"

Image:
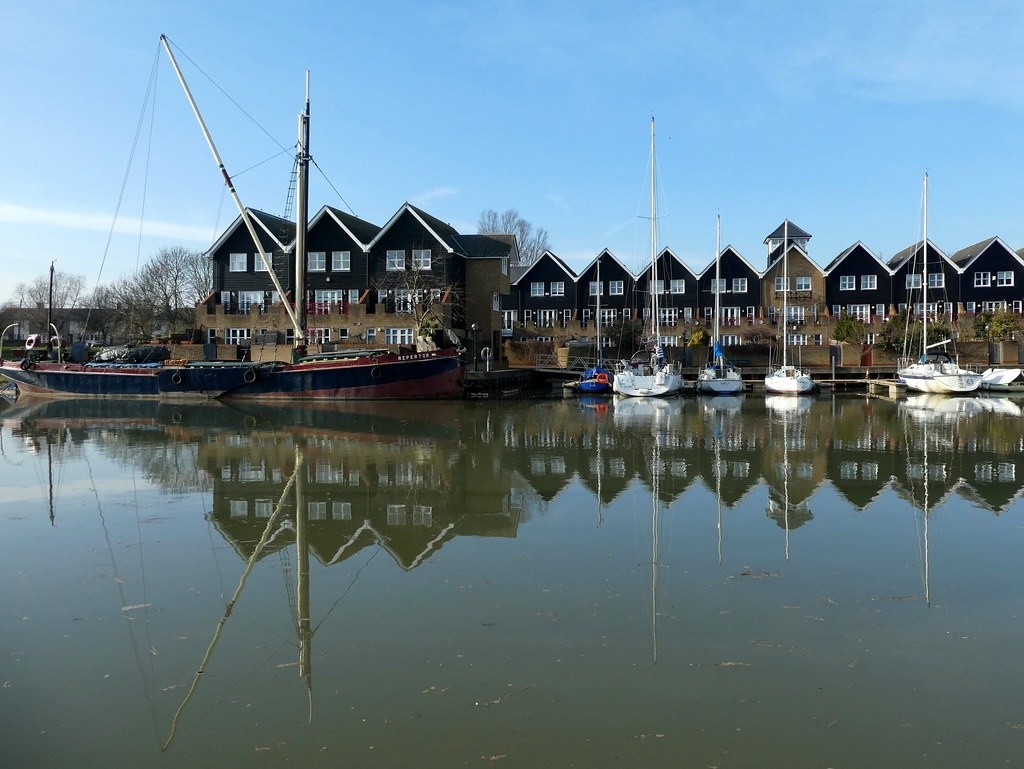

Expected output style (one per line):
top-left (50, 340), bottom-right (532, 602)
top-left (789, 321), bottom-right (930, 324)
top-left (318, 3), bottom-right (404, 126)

top-left (981, 366), bottom-right (1024, 393)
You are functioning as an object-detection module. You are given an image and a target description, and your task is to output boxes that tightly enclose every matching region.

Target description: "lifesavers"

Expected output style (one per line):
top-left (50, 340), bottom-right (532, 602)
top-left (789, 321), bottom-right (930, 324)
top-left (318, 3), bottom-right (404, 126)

top-left (171, 374), bottom-right (182, 384)
top-left (481, 347), bottom-right (494, 360)
top-left (244, 415), bottom-right (257, 430)
top-left (370, 367), bottom-right (382, 378)
top-left (244, 369), bottom-right (256, 384)
top-left (50, 337), bottom-right (65, 350)
top-left (171, 411), bottom-right (183, 424)
top-left (596, 402), bottom-right (608, 415)
top-left (25, 336), bottom-right (37, 349)
top-left (597, 373), bottom-right (607, 384)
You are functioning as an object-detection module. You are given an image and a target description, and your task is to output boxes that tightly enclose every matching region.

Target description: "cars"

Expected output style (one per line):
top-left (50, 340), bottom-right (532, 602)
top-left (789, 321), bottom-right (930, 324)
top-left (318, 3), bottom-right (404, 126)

top-left (85, 340), bottom-right (103, 347)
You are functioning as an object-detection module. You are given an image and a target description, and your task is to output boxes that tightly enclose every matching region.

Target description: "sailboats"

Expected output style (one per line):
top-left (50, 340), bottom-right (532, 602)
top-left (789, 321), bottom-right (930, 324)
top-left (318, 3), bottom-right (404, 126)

top-left (897, 172), bottom-right (984, 394)
top-left (695, 213), bottom-right (746, 395)
top-left (609, 115), bottom-right (684, 399)
top-left (762, 216), bottom-right (816, 395)
top-left (562, 260), bottom-right (614, 393)
top-left (0, 32), bottom-right (470, 402)
